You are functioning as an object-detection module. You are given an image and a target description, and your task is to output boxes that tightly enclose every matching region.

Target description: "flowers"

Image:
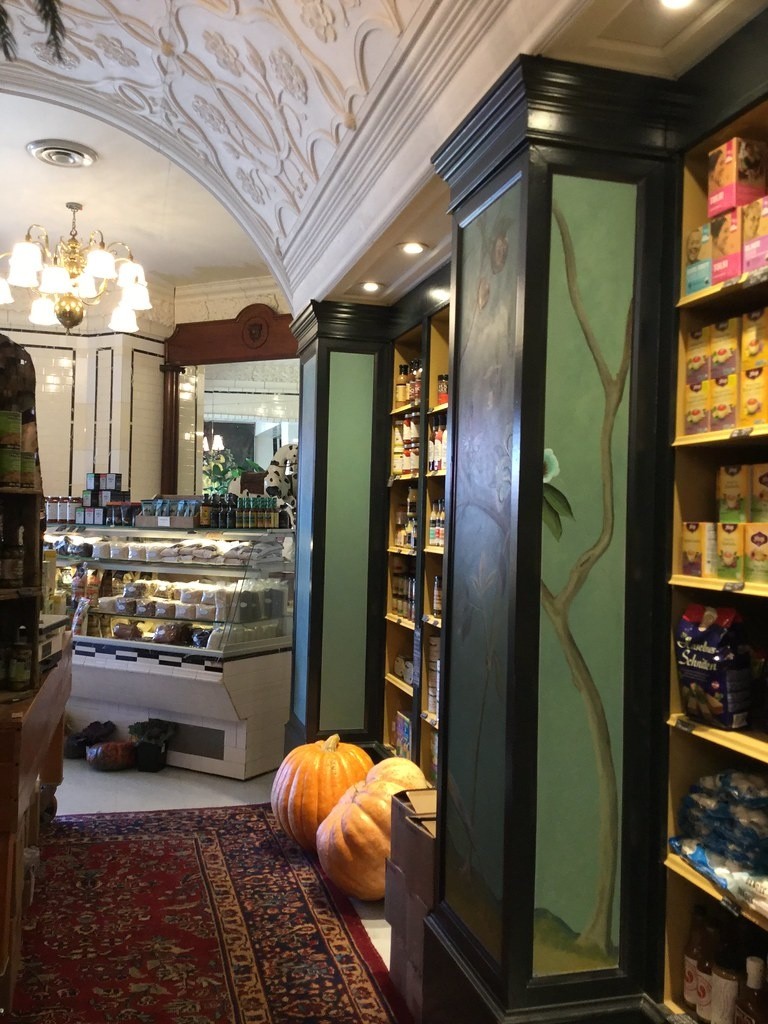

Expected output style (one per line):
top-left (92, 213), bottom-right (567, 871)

top-left (202, 448), bottom-right (242, 498)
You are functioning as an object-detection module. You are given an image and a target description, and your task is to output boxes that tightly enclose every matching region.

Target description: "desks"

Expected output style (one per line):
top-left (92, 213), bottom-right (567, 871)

top-left (0, 630), bottom-right (75, 1024)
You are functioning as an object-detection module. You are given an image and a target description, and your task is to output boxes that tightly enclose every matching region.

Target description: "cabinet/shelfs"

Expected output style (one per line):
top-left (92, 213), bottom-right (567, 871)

top-left (382, 301), bottom-right (452, 789)
top-left (40, 530), bottom-right (298, 782)
top-left (655, 99), bottom-right (768, 1021)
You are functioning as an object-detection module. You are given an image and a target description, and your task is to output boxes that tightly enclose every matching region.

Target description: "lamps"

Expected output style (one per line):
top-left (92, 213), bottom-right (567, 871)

top-left (28, 141), bottom-right (95, 169)
top-left (0, 203), bottom-right (153, 335)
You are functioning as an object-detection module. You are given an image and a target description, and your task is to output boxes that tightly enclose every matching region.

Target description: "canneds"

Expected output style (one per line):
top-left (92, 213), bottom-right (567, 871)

top-left (438, 374), bottom-right (449, 404)
top-left (390, 553), bottom-right (416, 625)
top-left (44, 496), bottom-right (83, 524)
top-left (0, 640), bottom-right (34, 690)
top-left (432, 575), bottom-right (442, 619)
top-left (0, 410), bottom-right (37, 488)
top-left (0, 509), bottom-right (26, 589)
top-left (391, 411), bottom-right (422, 475)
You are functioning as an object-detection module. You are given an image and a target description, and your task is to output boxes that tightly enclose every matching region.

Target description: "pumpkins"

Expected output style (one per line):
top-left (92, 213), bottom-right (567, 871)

top-left (87, 739), bottom-right (135, 771)
top-left (271, 733), bottom-right (374, 850)
top-left (316, 757), bottom-right (430, 902)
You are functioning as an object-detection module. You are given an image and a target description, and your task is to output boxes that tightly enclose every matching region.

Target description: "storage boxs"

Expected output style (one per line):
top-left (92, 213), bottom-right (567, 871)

top-left (0, 809), bottom-right (34, 1013)
top-left (75, 472), bottom-right (130, 525)
top-left (384, 791), bottom-right (438, 1022)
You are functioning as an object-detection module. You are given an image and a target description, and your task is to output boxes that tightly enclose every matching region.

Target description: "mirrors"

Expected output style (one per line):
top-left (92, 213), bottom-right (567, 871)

top-left (161, 305), bottom-right (301, 500)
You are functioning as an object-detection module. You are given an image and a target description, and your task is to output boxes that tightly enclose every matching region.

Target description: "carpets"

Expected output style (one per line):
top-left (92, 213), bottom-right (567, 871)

top-left (0, 802), bottom-right (415, 1024)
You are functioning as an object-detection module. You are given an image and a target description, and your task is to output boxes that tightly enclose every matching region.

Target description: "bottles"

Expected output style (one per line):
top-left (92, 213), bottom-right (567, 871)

top-left (433, 576), bottom-right (443, 619)
top-left (428, 414), bottom-right (447, 470)
top-left (396, 360), bottom-right (421, 408)
top-left (199, 492), bottom-right (279, 528)
top-left (682, 902), bottom-right (768, 1024)
top-left (438, 374), bottom-right (449, 405)
top-left (430, 498), bottom-right (444, 546)
top-left (395, 486), bottom-right (416, 548)
